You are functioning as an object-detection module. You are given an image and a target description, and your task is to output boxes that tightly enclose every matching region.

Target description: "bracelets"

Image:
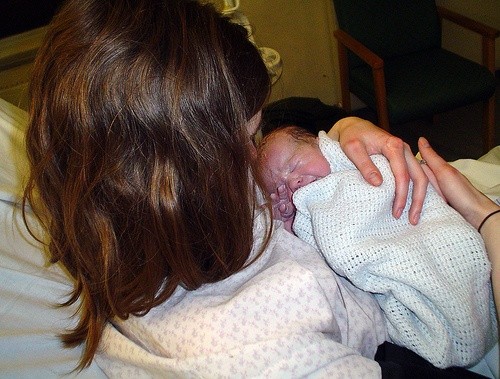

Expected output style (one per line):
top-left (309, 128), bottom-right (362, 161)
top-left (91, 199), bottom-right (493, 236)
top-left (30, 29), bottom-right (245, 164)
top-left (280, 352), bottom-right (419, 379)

top-left (477, 210), bottom-right (500, 233)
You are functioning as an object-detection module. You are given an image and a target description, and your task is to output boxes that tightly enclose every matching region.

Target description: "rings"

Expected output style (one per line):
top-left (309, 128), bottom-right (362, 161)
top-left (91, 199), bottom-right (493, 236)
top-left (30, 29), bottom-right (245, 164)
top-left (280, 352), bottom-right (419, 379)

top-left (419, 160), bottom-right (428, 165)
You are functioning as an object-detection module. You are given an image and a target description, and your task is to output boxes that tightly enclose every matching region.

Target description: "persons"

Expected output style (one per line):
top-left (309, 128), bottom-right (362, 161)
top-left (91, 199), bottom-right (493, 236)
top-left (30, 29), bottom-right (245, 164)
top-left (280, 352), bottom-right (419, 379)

top-left (251, 123), bottom-right (499, 370)
top-left (21, 0), bottom-right (500, 379)
